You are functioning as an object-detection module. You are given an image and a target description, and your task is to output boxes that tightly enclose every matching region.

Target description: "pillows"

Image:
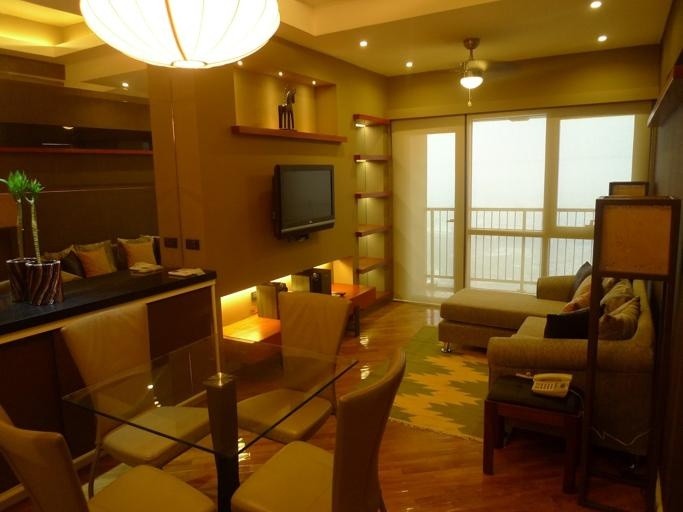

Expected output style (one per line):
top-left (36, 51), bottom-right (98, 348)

top-left (45, 237), bottom-right (157, 283)
top-left (542, 261), bottom-right (639, 340)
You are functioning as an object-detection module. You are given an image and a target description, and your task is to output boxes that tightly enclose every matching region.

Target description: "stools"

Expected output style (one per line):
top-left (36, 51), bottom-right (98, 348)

top-left (482, 375), bottom-right (582, 494)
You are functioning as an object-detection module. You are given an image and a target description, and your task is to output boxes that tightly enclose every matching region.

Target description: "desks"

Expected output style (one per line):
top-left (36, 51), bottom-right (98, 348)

top-left (1, 290), bottom-right (407, 512)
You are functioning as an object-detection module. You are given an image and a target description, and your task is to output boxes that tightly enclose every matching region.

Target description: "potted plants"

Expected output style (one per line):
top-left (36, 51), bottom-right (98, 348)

top-left (0, 169), bottom-right (36, 303)
top-left (22, 175), bottom-right (65, 305)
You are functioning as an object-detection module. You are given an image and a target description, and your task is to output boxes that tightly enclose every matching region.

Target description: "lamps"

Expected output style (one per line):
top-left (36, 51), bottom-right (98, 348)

top-left (577, 194), bottom-right (682, 512)
top-left (458, 39), bottom-right (484, 108)
top-left (78, 0), bottom-right (280, 71)
top-left (609, 180), bottom-right (650, 195)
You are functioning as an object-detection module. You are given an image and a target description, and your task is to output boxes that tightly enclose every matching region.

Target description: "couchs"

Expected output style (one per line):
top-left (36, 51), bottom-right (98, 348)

top-left (437, 276), bottom-right (655, 488)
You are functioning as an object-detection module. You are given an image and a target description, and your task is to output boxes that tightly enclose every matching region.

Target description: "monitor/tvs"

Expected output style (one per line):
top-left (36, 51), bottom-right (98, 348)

top-left (275, 164), bottom-right (335, 240)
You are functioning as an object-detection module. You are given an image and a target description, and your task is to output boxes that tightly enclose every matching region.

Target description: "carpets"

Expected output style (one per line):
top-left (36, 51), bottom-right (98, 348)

top-left (358, 324), bottom-right (490, 443)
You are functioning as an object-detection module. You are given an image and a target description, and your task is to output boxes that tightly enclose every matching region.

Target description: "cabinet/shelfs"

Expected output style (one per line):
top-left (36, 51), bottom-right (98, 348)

top-left (354, 114), bottom-right (393, 311)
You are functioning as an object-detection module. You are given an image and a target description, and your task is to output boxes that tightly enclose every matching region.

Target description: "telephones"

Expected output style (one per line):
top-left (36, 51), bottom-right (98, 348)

top-left (531, 373), bottom-right (573, 398)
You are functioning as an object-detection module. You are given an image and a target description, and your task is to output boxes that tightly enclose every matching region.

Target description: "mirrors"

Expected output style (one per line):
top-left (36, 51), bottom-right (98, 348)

top-left (0, 48), bottom-right (184, 308)
